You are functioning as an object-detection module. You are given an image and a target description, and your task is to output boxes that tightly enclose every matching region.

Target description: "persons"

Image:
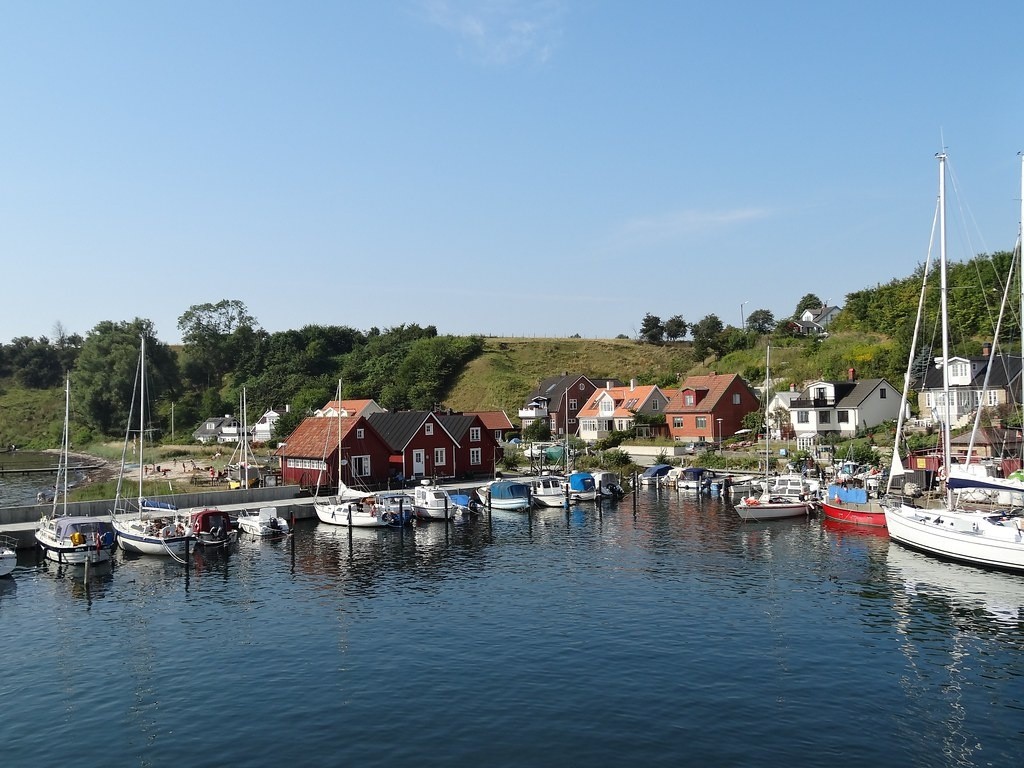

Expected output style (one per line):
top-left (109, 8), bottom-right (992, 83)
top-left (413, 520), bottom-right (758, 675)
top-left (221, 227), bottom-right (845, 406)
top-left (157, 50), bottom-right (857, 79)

top-left (182, 463), bottom-right (187, 472)
top-left (144, 463), bottom-right (167, 476)
top-left (172, 458), bottom-right (176, 466)
top-left (189, 460), bottom-right (197, 470)
top-left (11, 443), bottom-right (16, 451)
top-left (209, 466), bottom-right (215, 477)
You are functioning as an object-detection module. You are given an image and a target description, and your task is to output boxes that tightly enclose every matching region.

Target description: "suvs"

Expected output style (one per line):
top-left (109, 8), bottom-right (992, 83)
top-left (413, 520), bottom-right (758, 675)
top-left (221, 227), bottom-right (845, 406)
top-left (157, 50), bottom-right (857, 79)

top-left (684, 442), bottom-right (716, 455)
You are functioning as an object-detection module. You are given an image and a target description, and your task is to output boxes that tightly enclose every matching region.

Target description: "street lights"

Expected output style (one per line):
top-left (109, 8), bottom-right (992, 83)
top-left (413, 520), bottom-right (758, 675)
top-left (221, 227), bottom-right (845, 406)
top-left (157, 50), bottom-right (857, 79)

top-left (716, 418), bottom-right (724, 456)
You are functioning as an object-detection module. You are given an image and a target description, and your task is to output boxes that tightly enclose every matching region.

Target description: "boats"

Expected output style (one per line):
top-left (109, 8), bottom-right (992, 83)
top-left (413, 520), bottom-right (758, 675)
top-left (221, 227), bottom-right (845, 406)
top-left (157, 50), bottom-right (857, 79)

top-left (819, 519), bottom-right (893, 563)
top-left (0, 534), bottom-right (19, 579)
top-left (590, 472), bottom-right (624, 497)
top-left (562, 472), bottom-right (597, 500)
top-left (412, 521), bottom-right (459, 567)
top-left (678, 468), bottom-right (716, 489)
top-left (475, 478), bottom-right (535, 511)
top-left (734, 471), bottom-right (821, 521)
top-left (379, 494), bottom-right (413, 523)
top-left (820, 483), bottom-right (888, 527)
top-left (886, 541), bottom-right (1024, 638)
top-left (412, 479), bottom-right (459, 520)
top-left (529, 476), bottom-right (568, 508)
top-left (232, 506), bottom-right (290, 537)
top-left (640, 464), bottom-right (675, 486)
top-left (190, 510), bottom-right (237, 548)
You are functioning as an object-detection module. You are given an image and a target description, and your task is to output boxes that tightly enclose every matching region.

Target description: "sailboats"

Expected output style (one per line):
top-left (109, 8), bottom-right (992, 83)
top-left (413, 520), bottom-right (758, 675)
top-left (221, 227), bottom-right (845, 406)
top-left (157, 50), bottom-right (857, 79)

top-left (880, 126), bottom-right (1023, 574)
top-left (313, 522), bottom-right (391, 587)
top-left (313, 378), bottom-right (391, 526)
top-left (109, 333), bottom-right (197, 557)
top-left (34, 370), bottom-right (111, 565)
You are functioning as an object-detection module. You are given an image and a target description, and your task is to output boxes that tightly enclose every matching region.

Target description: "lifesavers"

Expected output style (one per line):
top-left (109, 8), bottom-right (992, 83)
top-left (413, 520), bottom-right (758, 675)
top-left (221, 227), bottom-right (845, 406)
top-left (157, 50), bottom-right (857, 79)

top-left (70, 533), bottom-right (83, 546)
